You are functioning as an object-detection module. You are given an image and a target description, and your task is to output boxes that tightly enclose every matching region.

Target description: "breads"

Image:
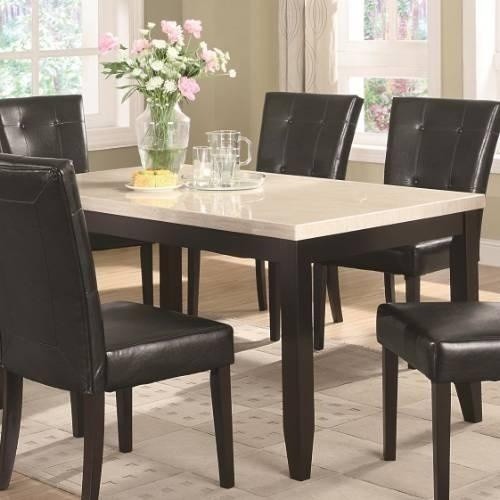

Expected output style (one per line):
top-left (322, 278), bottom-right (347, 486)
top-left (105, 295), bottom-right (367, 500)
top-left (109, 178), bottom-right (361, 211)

top-left (133, 170), bottom-right (176, 188)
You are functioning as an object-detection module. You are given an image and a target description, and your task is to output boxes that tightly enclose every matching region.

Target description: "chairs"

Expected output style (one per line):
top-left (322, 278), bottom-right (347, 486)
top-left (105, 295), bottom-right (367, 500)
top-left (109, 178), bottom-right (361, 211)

top-left (2, 93), bottom-right (155, 308)
top-left (376, 300), bottom-right (500, 498)
top-left (187, 92), bottom-right (364, 340)
top-left (1, 151), bottom-right (235, 498)
top-left (312, 97), bottom-right (500, 371)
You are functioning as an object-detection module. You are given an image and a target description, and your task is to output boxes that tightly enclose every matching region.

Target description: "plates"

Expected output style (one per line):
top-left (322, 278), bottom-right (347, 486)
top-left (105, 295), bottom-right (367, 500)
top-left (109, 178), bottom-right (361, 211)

top-left (185, 169), bottom-right (266, 191)
top-left (123, 177), bottom-right (186, 196)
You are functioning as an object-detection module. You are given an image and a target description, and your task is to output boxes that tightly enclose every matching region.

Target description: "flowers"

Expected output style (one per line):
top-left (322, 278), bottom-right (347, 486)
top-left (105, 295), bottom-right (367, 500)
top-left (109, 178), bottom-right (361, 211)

top-left (95, 19), bottom-right (237, 169)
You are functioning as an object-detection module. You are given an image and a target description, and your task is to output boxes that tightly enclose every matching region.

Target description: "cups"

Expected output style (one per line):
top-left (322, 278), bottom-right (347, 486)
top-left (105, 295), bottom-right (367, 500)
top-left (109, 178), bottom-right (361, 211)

top-left (192, 143), bottom-right (213, 188)
top-left (206, 129), bottom-right (253, 188)
